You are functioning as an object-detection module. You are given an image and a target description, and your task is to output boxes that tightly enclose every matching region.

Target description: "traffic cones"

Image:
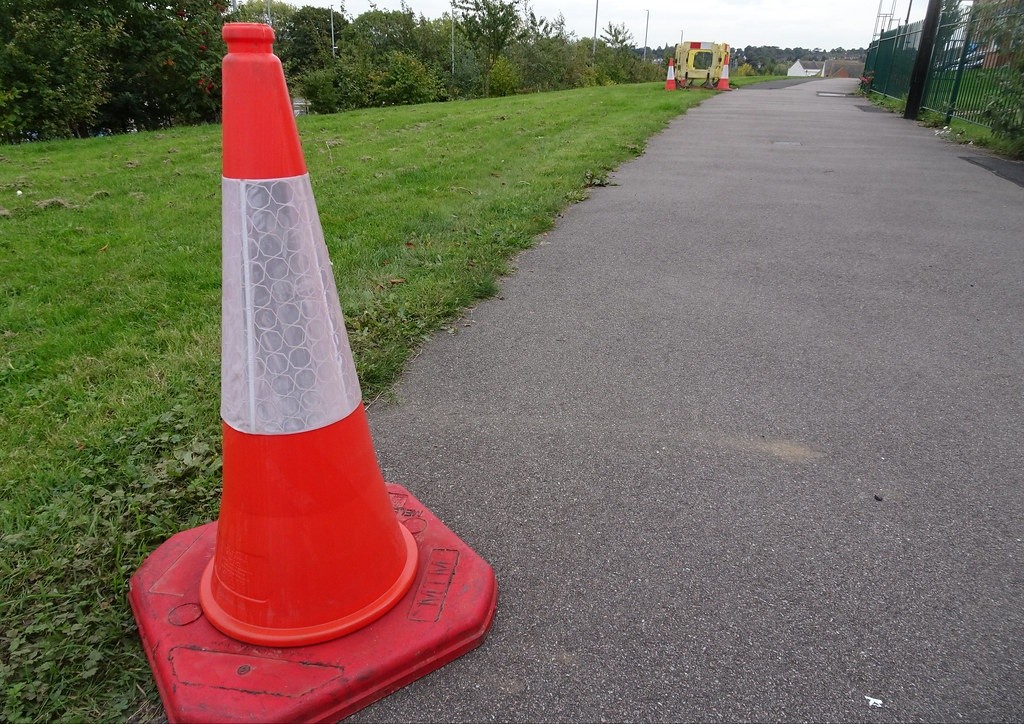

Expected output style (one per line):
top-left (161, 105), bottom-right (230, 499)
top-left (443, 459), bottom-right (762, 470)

top-left (124, 21), bottom-right (500, 724)
top-left (716, 56), bottom-right (731, 91)
top-left (665, 58), bottom-right (677, 90)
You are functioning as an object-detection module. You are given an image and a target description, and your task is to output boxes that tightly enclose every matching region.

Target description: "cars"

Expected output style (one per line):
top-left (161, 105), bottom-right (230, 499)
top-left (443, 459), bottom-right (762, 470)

top-left (934, 50), bottom-right (986, 71)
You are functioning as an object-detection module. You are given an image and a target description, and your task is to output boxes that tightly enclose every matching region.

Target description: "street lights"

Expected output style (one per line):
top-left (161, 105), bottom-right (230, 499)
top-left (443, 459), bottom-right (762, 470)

top-left (643, 10), bottom-right (649, 62)
top-left (680, 30), bottom-right (683, 44)
